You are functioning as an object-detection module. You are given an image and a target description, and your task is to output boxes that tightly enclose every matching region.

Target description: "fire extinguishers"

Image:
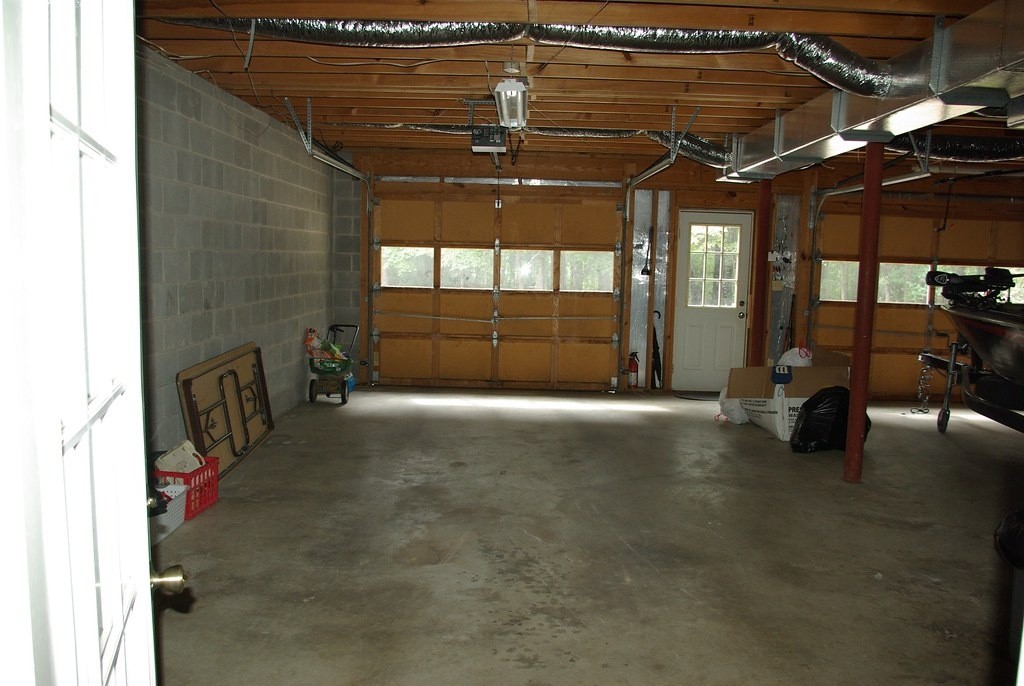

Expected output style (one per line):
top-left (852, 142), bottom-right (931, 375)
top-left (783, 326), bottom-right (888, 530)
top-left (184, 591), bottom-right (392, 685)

top-left (629, 352), bottom-right (641, 388)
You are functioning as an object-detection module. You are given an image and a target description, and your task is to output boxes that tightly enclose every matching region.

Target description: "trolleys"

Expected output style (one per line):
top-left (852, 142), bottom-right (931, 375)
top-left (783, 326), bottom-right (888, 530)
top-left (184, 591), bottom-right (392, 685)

top-left (304, 324), bottom-right (359, 404)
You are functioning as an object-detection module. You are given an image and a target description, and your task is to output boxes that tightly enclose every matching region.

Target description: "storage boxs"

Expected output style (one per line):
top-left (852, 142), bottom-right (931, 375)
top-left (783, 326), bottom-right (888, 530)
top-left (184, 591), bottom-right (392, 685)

top-left (726, 349), bottom-right (850, 441)
top-left (150, 484), bottom-right (189, 547)
top-left (152, 456), bottom-right (219, 523)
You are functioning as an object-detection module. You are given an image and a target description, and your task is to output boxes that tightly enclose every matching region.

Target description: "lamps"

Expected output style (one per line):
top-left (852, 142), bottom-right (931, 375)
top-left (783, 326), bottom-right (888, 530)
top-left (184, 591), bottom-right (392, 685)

top-left (493, 78), bottom-right (527, 129)
